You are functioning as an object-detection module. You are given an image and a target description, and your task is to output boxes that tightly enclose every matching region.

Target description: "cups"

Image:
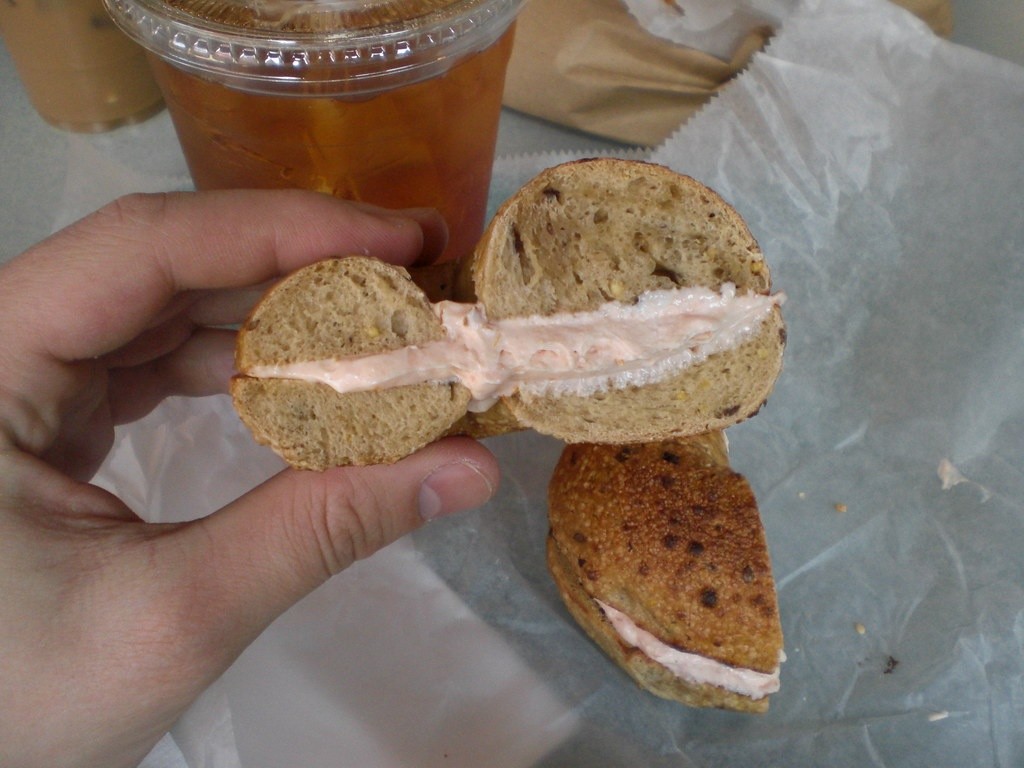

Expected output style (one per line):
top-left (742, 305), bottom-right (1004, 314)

top-left (0, 0), bottom-right (166, 136)
top-left (99, 1), bottom-right (532, 312)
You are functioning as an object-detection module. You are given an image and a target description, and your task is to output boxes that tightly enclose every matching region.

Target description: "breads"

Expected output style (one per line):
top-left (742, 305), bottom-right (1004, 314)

top-left (228, 155), bottom-right (785, 474)
top-left (545, 430), bottom-right (787, 716)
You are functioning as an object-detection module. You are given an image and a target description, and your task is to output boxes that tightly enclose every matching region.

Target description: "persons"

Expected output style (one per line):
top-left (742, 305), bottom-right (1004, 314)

top-left (0, 187), bottom-right (498, 768)
